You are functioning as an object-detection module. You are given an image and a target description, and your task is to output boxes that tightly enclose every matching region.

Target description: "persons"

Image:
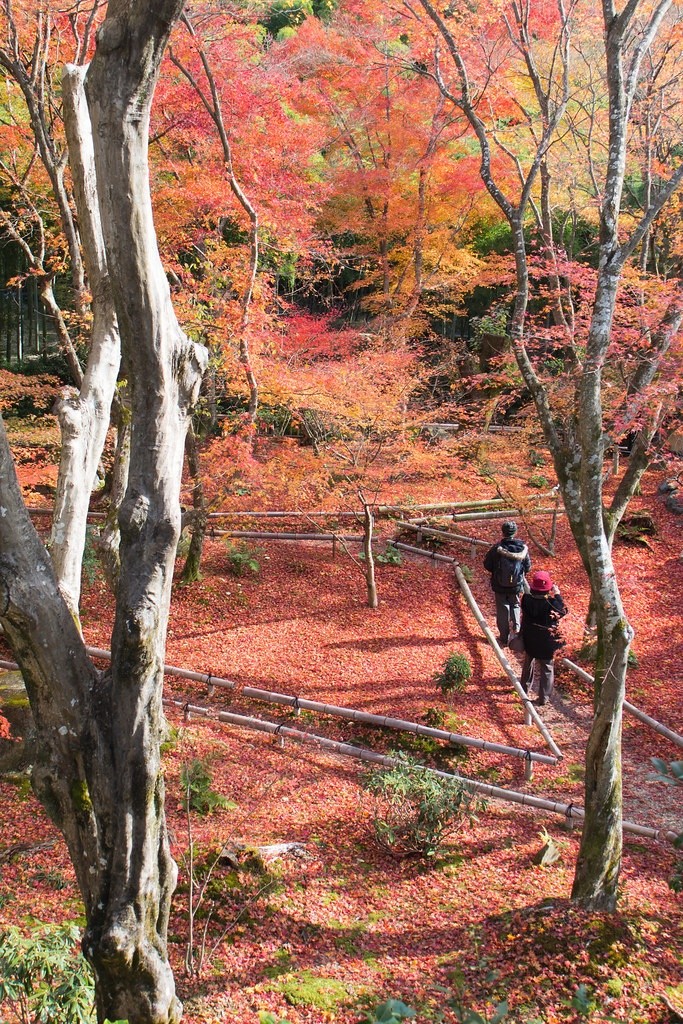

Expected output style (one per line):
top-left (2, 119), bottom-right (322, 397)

top-left (521, 571), bottom-right (568, 706)
top-left (483, 521), bottom-right (531, 642)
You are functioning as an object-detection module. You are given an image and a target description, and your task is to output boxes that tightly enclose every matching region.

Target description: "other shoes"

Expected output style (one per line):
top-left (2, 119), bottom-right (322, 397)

top-left (540, 699), bottom-right (547, 705)
top-left (498, 638), bottom-right (508, 646)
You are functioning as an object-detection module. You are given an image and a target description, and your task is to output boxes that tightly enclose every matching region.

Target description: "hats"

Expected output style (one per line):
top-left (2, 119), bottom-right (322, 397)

top-left (530, 571), bottom-right (553, 591)
top-left (502, 521), bottom-right (517, 534)
